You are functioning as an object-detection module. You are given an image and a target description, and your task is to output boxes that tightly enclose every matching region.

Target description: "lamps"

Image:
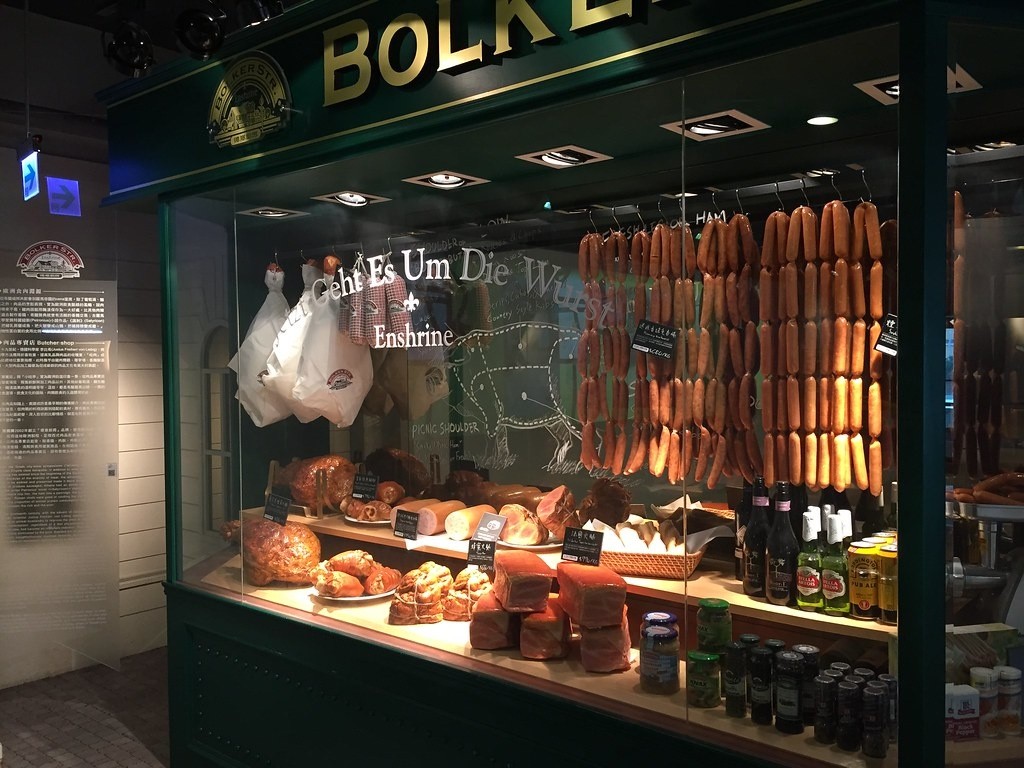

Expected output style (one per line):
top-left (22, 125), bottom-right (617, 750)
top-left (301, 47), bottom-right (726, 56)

top-left (175, 0), bottom-right (228, 61)
top-left (101, 0), bottom-right (153, 78)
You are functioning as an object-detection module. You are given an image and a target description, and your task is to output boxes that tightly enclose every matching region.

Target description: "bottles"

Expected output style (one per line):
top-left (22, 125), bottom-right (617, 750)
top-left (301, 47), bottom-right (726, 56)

top-left (724, 633), bottom-right (898, 758)
top-left (735, 476), bottom-right (897, 616)
top-left (969, 665), bottom-right (1023, 739)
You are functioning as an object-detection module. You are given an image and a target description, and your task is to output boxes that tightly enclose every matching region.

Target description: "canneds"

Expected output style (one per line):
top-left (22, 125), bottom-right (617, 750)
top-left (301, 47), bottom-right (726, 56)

top-left (847, 529), bottom-right (897, 628)
top-left (641, 611), bottom-right (681, 695)
top-left (970, 666), bottom-right (1022, 736)
top-left (687, 597), bottom-right (896, 758)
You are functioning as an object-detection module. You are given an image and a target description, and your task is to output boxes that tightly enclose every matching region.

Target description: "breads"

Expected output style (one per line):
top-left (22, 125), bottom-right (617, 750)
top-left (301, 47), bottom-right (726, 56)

top-left (597, 517), bottom-right (689, 554)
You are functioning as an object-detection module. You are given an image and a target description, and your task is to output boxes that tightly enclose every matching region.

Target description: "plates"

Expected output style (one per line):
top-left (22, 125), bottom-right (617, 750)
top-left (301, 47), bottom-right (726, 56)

top-left (345, 515), bottom-right (391, 523)
top-left (311, 586), bottom-right (396, 601)
top-left (496, 537), bottom-right (563, 551)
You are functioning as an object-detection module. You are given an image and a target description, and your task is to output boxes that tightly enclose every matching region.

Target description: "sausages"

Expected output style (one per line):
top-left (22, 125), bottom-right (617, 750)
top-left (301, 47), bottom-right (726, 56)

top-left (945, 472), bottom-right (1024, 506)
top-left (388, 485), bottom-right (551, 540)
top-left (954, 190), bottom-right (1002, 476)
top-left (576, 200), bottom-right (897, 498)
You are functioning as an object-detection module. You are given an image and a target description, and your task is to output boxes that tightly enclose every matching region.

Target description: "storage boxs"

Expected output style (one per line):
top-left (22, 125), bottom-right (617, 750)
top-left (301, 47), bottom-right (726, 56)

top-left (888, 622), bottom-right (1018, 685)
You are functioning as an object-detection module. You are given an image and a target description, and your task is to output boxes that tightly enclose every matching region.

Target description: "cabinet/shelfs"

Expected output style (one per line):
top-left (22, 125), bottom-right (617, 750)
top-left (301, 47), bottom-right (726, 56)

top-left (94, 0), bottom-right (1024, 768)
